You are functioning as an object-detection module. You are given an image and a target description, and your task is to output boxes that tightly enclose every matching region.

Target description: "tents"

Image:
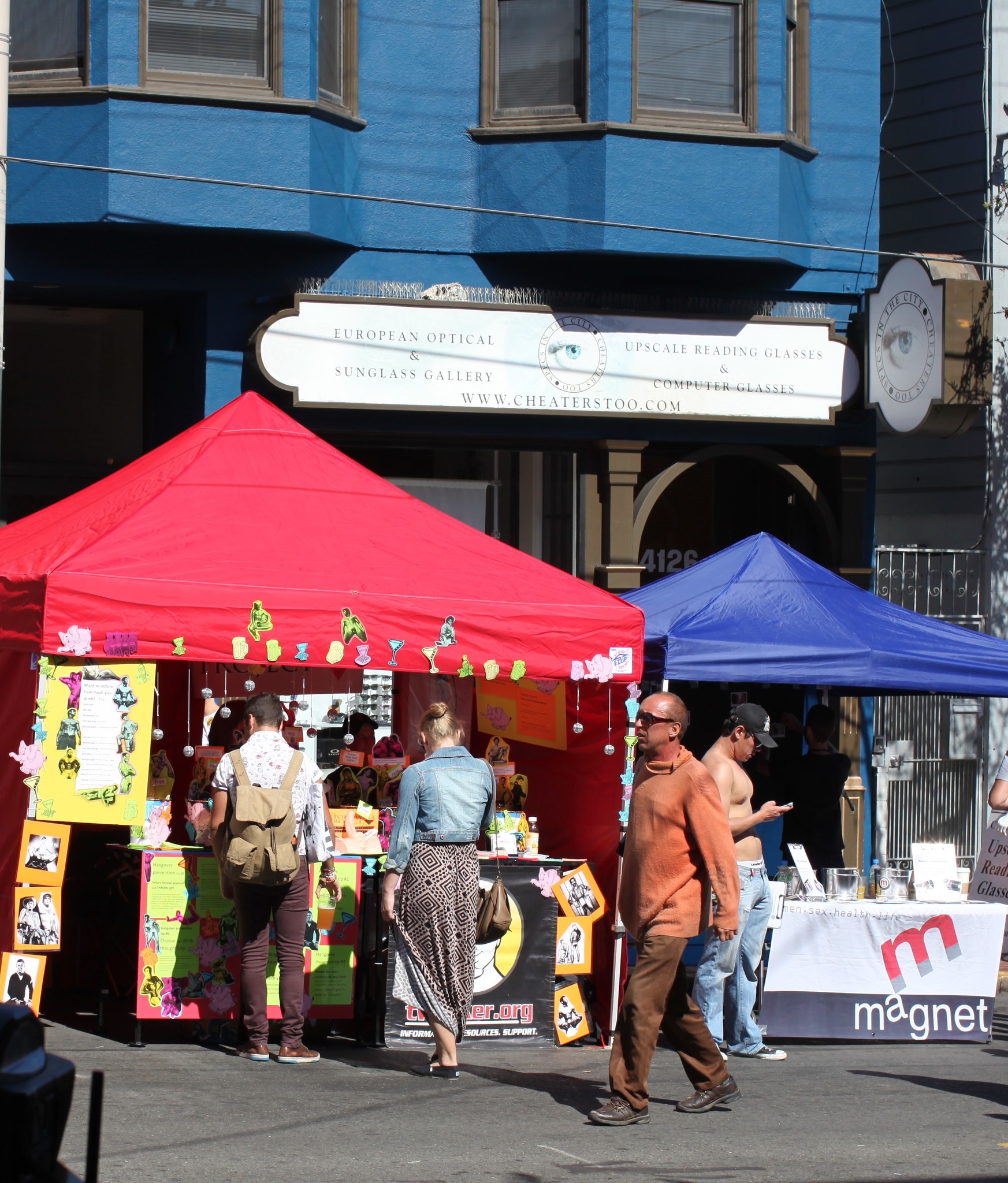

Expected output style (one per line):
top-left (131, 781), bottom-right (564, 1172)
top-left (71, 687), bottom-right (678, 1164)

top-left (619, 531), bottom-right (1008, 697)
top-left (0, 391), bottom-right (645, 1050)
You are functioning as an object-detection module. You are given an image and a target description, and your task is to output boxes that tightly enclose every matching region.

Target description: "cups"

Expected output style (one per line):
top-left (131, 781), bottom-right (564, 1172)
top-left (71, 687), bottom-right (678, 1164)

top-left (956, 867), bottom-right (970, 901)
top-left (858, 876), bottom-right (867, 899)
top-left (317, 896), bottom-right (337, 930)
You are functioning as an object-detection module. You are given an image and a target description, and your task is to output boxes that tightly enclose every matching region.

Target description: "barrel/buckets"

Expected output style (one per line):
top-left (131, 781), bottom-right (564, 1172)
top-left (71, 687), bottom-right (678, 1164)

top-left (875, 868), bottom-right (913, 901)
top-left (773, 866), bottom-right (805, 900)
top-left (821, 867), bottom-right (863, 901)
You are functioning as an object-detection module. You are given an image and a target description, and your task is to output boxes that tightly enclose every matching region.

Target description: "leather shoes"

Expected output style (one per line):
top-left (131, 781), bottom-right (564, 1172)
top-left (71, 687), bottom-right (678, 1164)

top-left (588, 1094), bottom-right (650, 1126)
top-left (675, 1075), bottom-right (741, 1112)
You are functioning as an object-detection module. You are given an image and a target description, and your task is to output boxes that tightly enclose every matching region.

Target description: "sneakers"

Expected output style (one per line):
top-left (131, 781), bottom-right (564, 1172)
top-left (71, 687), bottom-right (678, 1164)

top-left (751, 1045), bottom-right (787, 1060)
top-left (717, 1048), bottom-right (728, 1061)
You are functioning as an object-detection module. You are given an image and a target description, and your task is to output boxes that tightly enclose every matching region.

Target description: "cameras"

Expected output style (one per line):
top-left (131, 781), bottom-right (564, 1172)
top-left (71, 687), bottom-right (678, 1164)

top-left (795, 726), bottom-right (807, 735)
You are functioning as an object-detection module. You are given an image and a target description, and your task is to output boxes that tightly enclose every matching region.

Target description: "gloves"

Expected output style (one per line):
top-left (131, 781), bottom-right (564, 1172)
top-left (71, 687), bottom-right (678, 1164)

top-left (995, 813), bottom-right (1008, 836)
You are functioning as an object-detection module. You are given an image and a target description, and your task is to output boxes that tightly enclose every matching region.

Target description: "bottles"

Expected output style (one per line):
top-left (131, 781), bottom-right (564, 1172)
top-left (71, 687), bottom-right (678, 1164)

top-left (868, 859), bottom-right (881, 898)
top-left (524, 817), bottom-right (539, 854)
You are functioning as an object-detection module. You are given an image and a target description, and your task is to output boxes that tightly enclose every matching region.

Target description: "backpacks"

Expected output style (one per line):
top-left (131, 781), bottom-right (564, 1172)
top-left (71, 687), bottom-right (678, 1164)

top-left (220, 748), bottom-right (303, 887)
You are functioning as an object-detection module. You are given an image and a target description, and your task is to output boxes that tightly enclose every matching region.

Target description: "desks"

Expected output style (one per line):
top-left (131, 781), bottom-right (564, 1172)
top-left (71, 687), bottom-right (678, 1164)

top-left (98, 841), bottom-right (586, 1050)
top-left (756, 899), bottom-right (1008, 1044)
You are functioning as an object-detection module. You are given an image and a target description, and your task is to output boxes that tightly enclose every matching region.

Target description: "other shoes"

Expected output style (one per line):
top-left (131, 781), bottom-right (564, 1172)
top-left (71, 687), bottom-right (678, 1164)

top-left (235, 1044), bottom-right (320, 1063)
top-left (409, 1054), bottom-right (460, 1080)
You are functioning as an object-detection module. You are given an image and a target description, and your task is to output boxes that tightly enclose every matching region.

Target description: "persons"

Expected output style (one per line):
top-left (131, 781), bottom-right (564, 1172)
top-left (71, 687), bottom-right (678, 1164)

top-left (750, 705), bottom-right (852, 882)
top-left (324, 713), bottom-right (379, 805)
top-left (589, 692), bottom-right (741, 1125)
top-left (988, 750), bottom-right (1008, 809)
top-left (382, 703), bottom-right (496, 1080)
top-left (205, 694), bottom-right (342, 1063)
top-left (692, 704), bottom-right (793, 1061)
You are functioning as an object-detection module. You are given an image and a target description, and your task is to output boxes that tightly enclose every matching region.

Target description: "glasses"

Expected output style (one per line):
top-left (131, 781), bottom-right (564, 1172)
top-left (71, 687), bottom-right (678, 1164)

top-left (747, 730), bottom-right (762, 748)
top-left (380, 705), bottom-right (390, 708)
top-left (378, 675), bottom-right (392, 680)
top-left (636, 711), bottom-right (677, 725)
top-left (358, 704), bottom-right (377, 710)
top-left (369, 715), bottom-right (391, 726)
top-left (294, 720), bottom-right (307, 725)
top-left (362, 684), bottom-right (377, 690)
top-left (379, 695), bottom-right (391, 698)
top-left (328, 699), bottom-right (339, 722)
top-left (378, 685), bottom-right (392, 689)
top-left (363, 675), bottom-right (378, 678)
top-left (360, 694), bottom-right (378, 701)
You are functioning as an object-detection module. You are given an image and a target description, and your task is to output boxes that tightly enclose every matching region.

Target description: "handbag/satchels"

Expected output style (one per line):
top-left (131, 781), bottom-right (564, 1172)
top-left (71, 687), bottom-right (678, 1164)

top-left (476, 879), bottom-right (512, 945)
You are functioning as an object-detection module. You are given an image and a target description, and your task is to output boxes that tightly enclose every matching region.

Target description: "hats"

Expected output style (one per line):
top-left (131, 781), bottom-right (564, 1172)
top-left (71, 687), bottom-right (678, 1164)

top-left (730, 703), bottom-right (778, 748)
top-left (341, 712), bottom-right (378, 733)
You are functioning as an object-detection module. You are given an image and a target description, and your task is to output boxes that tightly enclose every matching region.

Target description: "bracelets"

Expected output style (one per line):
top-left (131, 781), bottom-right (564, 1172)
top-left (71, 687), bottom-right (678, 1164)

top-left (319, 867), bottom-right (337, 883)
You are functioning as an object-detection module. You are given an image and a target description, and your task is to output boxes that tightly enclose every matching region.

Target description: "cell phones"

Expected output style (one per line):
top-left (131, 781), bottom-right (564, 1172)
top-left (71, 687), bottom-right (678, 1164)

top-left (777, 801), bottom-right (794, 808)
